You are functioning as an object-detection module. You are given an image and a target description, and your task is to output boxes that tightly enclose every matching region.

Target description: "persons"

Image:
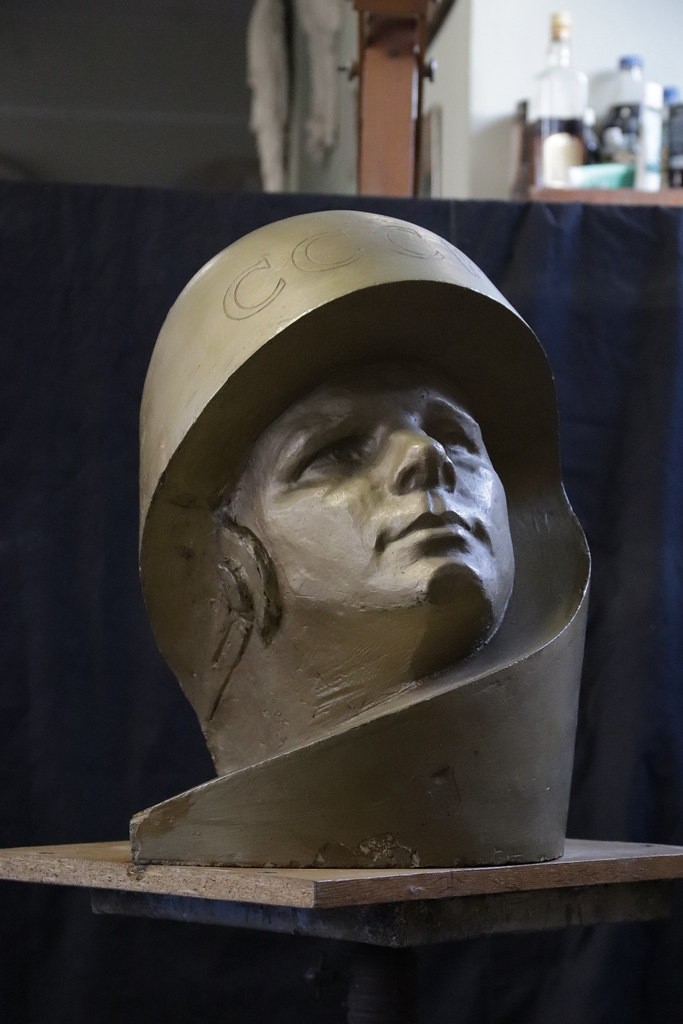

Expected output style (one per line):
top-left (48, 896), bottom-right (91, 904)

top-left (127, 210), bottom-right (592, 870)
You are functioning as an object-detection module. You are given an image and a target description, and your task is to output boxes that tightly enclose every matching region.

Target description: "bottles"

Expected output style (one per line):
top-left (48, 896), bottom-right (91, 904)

top-left (518, 5), bottom-right (682, 200)
